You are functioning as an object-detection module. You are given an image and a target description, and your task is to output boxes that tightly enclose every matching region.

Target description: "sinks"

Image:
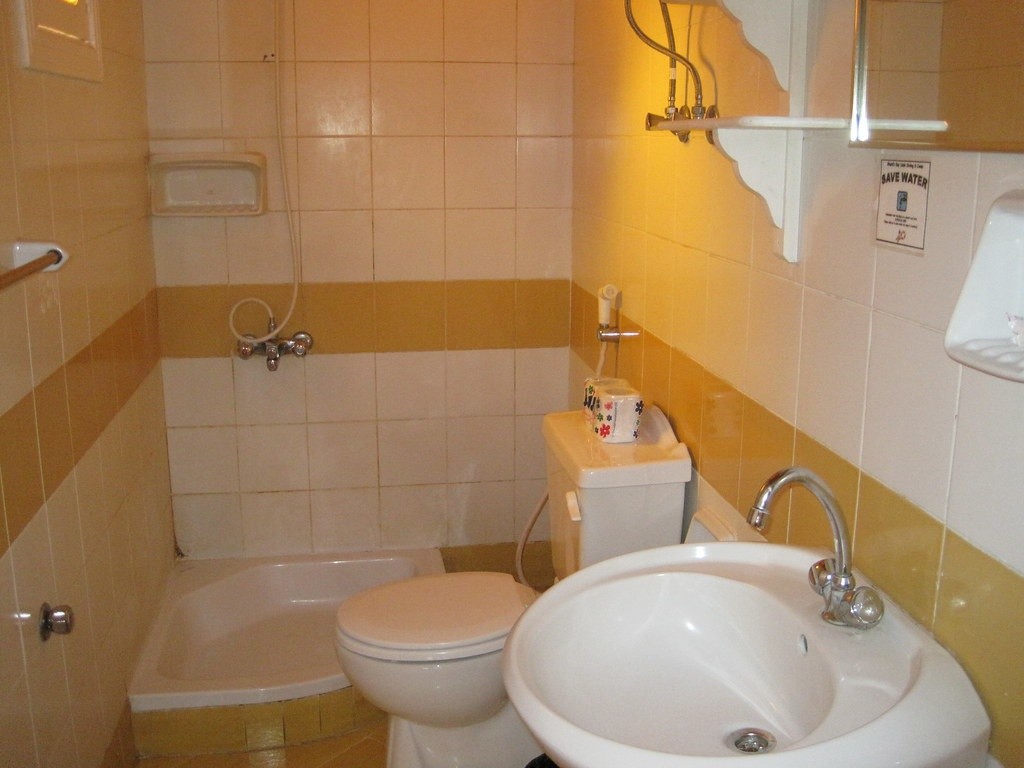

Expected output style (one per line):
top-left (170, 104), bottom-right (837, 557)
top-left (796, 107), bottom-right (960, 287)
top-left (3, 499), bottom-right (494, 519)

top-left (500, 541), bottom-right (991, 767)
top-left (128, 549), bottom-right (450, 712)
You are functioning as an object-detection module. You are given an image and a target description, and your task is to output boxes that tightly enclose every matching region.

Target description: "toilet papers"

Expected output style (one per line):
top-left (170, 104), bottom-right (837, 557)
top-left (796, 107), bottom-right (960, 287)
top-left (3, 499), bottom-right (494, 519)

top-left (584, 375), bottom-right (646, 445)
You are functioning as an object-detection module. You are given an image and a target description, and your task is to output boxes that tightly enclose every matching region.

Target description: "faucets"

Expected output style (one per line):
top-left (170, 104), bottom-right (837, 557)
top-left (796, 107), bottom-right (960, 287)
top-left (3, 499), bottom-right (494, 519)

top-left (746, 455), bottom-right (857, 615)
top-left (265, 315), bottom-right (283, 373)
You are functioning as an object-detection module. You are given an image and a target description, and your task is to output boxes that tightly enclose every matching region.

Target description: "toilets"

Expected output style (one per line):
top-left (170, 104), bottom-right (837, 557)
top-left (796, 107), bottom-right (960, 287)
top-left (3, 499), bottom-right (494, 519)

top-left (332, 385), bottom-right (692, 768)
